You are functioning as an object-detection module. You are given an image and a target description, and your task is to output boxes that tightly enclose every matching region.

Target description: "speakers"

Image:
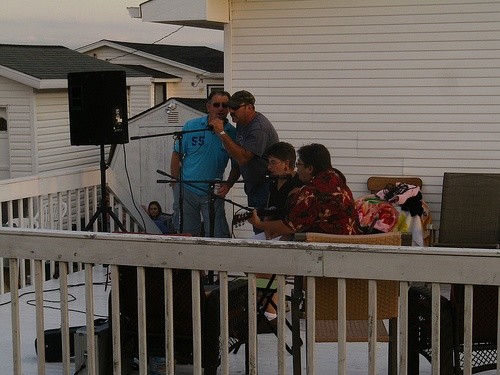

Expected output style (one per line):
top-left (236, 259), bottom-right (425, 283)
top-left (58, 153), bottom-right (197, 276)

top-left (67, 70), bottom-right (128, 145)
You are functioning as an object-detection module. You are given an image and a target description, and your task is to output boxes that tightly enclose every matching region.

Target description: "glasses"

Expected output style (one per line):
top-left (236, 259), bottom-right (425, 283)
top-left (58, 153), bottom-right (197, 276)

top-left (296, 162), bottom-right (304, 166)
top-left (207, 102), bottom-right (228, 109)
top-left (266, 161), bottom-right (285, 166)
top-left (229, 103), bottom-right (250, 111)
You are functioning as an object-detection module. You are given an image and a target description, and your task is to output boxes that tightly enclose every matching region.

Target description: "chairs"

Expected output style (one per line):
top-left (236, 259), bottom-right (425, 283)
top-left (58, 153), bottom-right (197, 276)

top-left (107, 232), bottom-right (305, 375)
top-left (366, 174), bottom-right (431, 245)
top-left (407, 171), bottom-right (500, 375)
top-left (291, 231), bottom-right (401, 375)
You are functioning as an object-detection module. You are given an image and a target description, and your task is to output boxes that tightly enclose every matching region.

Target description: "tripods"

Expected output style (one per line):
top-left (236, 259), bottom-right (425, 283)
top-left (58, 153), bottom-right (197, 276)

top-left (82, 146), bottom-right (127, 233)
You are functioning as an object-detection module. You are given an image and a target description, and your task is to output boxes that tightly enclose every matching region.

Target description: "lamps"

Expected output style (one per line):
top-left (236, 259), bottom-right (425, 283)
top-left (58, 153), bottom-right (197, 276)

top-left (165, 104), bottom-right (178, 115)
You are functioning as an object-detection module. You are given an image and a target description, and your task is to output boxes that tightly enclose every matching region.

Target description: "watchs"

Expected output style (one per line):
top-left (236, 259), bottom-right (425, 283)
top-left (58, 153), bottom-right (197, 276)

top-left (217, 130), bottom-right (227, 136)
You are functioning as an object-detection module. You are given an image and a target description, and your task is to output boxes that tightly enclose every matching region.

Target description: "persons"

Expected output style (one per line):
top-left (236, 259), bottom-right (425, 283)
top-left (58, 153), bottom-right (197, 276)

top-left (263, 141), bottom-right (304, 221)
top-left (248, 143), bottom-right (364, 321)
top-left (148, 200), bottom-right (177, 234)
top-left (170, 89), bottom-right (241, 237)
top-left (209, 90), bottom-right (280, 236)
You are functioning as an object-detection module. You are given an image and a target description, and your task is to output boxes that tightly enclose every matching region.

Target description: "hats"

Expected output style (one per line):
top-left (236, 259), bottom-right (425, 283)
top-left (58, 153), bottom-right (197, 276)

top-left (224, 90), bottom-right (256, 108)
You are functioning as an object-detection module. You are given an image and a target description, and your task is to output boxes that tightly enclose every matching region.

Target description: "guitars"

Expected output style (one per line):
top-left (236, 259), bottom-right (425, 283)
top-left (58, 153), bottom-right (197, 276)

top-left (232, 192), bottom-right (294, 228)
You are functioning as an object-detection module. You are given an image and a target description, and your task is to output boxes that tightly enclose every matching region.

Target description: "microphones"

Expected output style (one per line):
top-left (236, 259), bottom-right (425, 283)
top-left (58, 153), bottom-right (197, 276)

top-left (264, 173), bottom-right (292, 179)
top-left (207, 119), bottom-right (228, 127)
